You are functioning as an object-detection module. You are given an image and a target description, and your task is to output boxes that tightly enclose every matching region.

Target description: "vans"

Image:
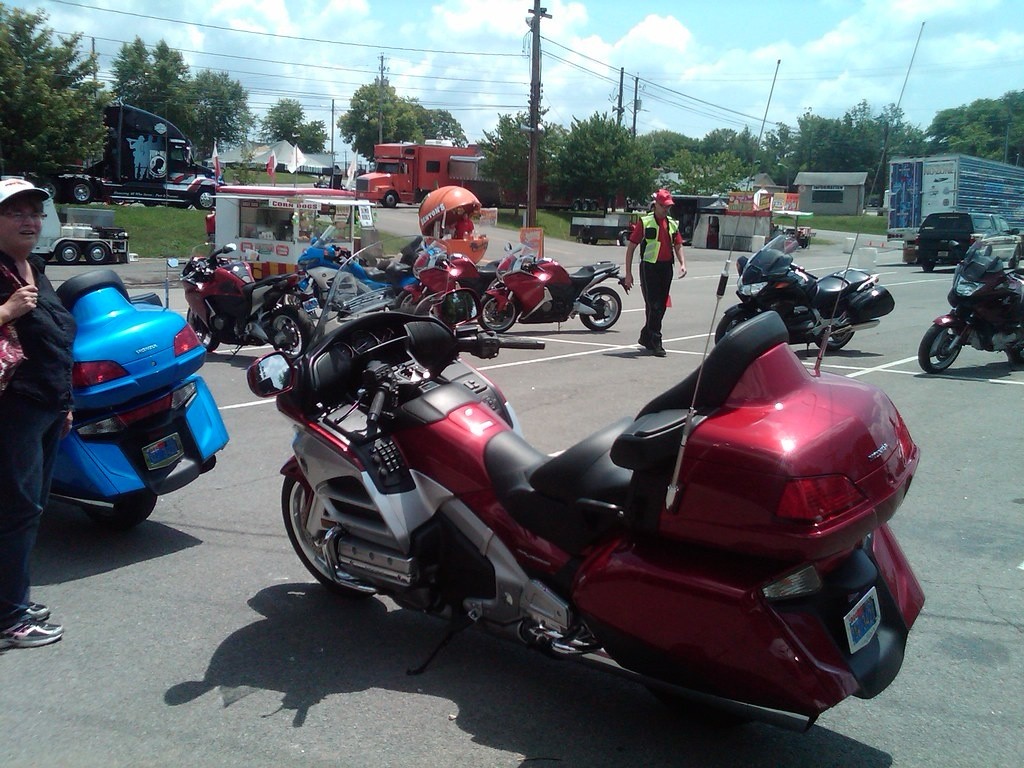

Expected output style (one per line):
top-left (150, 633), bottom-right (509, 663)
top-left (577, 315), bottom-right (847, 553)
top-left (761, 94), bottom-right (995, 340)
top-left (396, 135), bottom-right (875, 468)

top-left (913, 210), bottom-right (1011, 274)
top-left (916, 212), bottom-right (1020, 273)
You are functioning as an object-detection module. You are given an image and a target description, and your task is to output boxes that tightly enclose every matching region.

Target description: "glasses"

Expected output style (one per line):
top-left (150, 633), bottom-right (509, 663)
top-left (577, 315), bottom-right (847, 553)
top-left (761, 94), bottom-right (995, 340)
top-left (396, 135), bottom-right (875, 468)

top-left (660, 204), bottom-right (671, 208)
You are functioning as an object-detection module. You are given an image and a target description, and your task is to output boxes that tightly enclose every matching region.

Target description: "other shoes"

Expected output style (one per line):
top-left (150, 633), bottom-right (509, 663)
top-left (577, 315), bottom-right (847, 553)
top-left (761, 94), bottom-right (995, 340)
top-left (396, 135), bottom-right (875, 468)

top-left (653, 346), bottom-right (666, 356)
top-left (638, 337), bottom-right (653, 351)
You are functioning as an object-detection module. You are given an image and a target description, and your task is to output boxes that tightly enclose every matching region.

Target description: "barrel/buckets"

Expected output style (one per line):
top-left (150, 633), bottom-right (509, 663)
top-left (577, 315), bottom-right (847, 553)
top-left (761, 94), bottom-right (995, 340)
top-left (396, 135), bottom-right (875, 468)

top-left (843, 237), bottom-right (854, 254)
top-left (858, 248), bottom-right (876, 271)
top-left (752, 236), bottom-right (765, 252)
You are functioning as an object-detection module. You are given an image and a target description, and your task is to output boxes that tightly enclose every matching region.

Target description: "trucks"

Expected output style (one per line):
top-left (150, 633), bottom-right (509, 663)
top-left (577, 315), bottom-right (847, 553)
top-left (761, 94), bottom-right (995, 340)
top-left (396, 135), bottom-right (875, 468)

top-left (90, 101), bottom-right (227, 210)
top-left (355, 140), bottom-right (480, 206)
top-left (886, 154), bottom-right (1024, 265)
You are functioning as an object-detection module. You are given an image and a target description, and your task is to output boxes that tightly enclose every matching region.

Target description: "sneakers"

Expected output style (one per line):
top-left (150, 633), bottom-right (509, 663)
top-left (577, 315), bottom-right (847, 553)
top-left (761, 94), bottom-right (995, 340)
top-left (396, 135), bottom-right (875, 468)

top-left (0, 612), bottom-right (64, 649)
top-left (23, 601), bottom-right (50, 621)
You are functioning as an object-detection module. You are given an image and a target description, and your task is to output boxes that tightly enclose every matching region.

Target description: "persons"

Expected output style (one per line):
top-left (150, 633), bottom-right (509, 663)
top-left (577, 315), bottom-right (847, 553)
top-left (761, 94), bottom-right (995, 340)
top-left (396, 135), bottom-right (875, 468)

top-left (626, 188), bottom-right (688, 356)
top-left (0, 177), bottom-right (64, 649)
top-left (452, 212), bottom-right (474, 239)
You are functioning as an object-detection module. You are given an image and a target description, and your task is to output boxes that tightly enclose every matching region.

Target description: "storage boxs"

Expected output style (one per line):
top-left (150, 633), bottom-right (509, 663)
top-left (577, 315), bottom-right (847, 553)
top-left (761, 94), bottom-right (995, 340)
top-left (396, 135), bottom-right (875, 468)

top-left (663, 341), bottom-right (920, 564)
top-left (850, 285), bottom-right (896, 324)
top-left (66, 286), bottom-right (208, 410)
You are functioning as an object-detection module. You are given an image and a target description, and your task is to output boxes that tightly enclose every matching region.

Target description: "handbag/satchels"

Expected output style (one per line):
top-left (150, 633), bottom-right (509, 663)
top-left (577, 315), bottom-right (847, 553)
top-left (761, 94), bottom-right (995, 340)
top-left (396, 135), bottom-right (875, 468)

top-left (0, 262), bottom-right (28, 391)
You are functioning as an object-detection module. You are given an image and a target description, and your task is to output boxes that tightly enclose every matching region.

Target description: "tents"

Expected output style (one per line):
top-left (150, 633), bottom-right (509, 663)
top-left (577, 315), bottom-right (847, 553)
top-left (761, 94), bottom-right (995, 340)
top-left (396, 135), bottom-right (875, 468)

top-left (205, 140), bottom-right (307, 178)
top-left (691, 199), bottom-right (772, 250)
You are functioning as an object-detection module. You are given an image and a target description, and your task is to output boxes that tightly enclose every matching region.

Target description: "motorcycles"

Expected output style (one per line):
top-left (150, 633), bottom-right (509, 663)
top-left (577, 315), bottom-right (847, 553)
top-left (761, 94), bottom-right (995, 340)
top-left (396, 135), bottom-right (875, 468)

top-left (245, 231), bottom-right (929, 734)
top-left (714, 234), bottom-right (896, 355)
top-left (43, 223), bottom-right (624, 533)
top-left (918, 229), bottom-right (1024, 375)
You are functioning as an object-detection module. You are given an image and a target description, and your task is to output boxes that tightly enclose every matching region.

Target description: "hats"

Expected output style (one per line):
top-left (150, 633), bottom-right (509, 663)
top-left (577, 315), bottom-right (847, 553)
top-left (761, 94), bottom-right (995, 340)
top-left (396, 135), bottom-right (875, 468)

top-left (656, 189), bottom-right (675, 207)
top-left (0, 178), bottom-right (49, 203)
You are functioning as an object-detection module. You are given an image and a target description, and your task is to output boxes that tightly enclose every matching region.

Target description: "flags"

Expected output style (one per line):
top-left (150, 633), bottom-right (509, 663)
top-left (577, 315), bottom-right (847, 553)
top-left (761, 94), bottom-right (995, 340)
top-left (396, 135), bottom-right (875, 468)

top-left (346, 150), bottom-right (359, 186)
top-left (267, 148), bottom-right (277, 176)
top-left (288, 145), bottom-right (305, 173)
top-left (212, 141), bottom-right (221, 181)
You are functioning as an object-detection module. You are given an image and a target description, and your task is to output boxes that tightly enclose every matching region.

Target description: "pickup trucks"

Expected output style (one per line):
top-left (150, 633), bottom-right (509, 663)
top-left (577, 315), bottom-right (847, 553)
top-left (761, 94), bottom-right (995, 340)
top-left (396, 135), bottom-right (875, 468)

top-left (30, 198), bottom-right (131, 265)
